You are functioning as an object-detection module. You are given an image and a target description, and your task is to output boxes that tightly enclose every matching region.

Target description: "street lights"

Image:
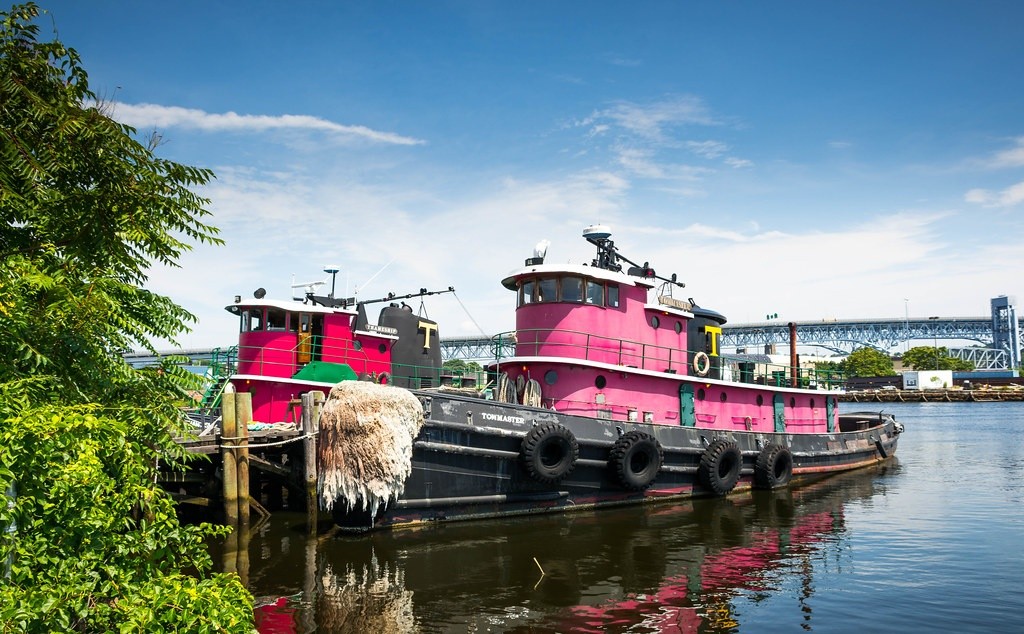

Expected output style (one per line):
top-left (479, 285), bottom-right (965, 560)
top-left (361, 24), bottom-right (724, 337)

top-left (929, 317), bottom-right (939, 370)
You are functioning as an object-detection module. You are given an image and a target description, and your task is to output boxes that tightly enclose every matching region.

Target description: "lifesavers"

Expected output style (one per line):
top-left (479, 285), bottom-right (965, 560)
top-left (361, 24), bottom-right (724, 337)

top-left (520, 419), bottom-right (578, 485)
top-left (694, 351), bottom-right (712, 377)
top-left (698, 438), bottom-right (742, 492)
top-left (757, 443), bottom-right (794, 487)
top-left (607, 431), bottom-right (664, 491)
top-left (528, 488), bottom-right (795, 616)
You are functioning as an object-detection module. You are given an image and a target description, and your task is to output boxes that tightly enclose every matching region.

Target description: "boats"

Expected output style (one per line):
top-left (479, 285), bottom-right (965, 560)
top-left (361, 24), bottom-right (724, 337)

top-left (196, 266), bottom-right (502, 425)
top-left (319, 227), bottom-right (904, 531)
